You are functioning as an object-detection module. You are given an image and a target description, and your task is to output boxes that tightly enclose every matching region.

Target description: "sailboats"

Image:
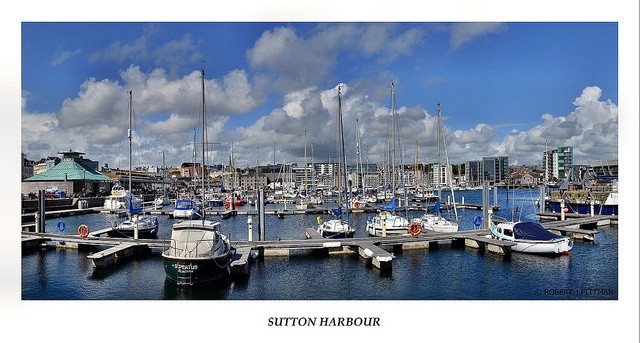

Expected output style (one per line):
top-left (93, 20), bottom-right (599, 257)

top-left (160, 69), bottom-right (235, 285)
top-left (224, 85), bottom-right (442, 205)
top-left (106, 89), bottom-right (159, 239)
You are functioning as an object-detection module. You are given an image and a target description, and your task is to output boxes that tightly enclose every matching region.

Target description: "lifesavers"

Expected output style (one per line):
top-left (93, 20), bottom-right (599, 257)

top-left (473, 214), bottom-right (482, 227)
top-left (77, 224), bottom-right (89, 238)
top-left (407, 222), bottom-right (422, 237)
top-left (224, 203), bottom-right (230, 210)
top-left (57, 221), bottom-right (66, 232)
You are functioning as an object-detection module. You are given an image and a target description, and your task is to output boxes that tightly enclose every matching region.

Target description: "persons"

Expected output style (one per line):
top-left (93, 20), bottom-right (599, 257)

top-left (588, 179), bottom-right (593, 192)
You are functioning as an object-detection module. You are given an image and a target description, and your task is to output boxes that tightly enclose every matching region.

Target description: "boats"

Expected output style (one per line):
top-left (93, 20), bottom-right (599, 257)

top-left (104, 184), bottom-right (143, 210)
top-left (366, 209), bottom-right (409, 237)
top-left (410, 213), bottom-right (460, 234)
top-left (488, 212), bottom-right (573, 254)
top-left (173, 198), bottom-right (202, 219)
top-left (535, 160), bottom-right (618, 215)
top-left (316, 217), bottom-right (355, 239)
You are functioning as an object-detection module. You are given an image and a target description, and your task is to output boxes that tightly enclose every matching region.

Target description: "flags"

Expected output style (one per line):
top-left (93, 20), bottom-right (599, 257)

top-left (384, 196), bottom-right (396, 211)
top-left (432, 198), bottom-right (440, 213)
top-left (388, 184), bottom-right (391, 190)
